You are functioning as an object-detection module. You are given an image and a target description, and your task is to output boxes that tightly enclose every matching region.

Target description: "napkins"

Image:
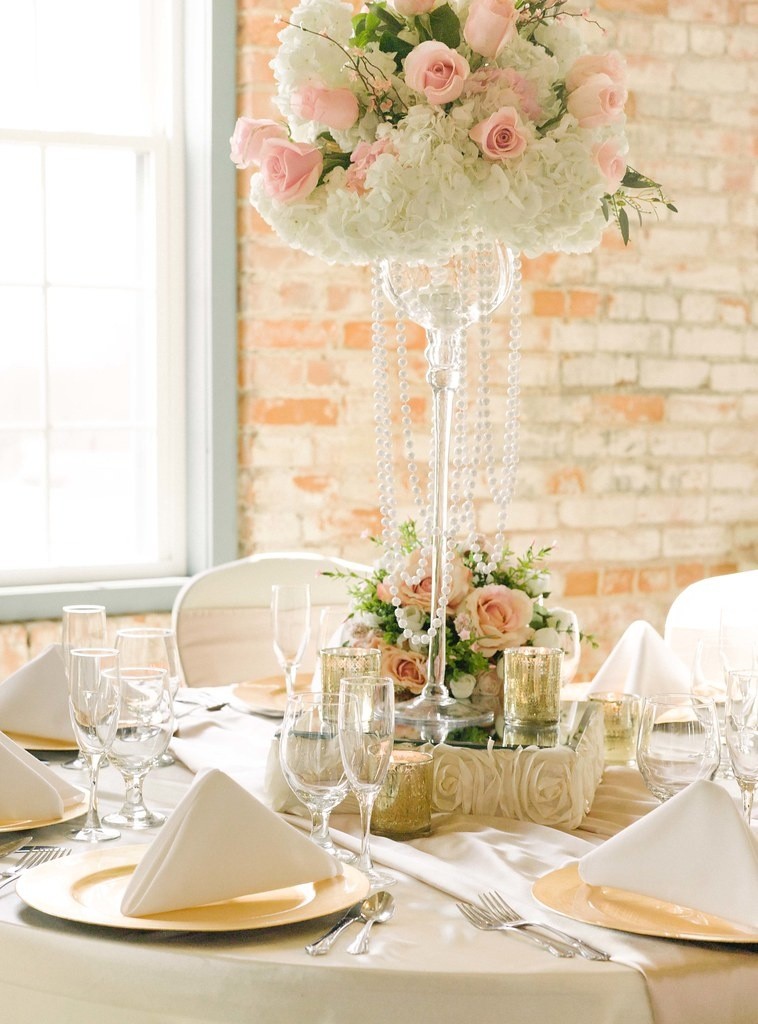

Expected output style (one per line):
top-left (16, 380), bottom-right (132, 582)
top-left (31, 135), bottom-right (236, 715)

top-left (0, 733), bottom-right (85, 823)
top-left (1, 642), bottom-right (103, 739)
top-left (588, 620), bottom-right (699, 707)
top-left (118, 768), bottom-right (341, 915)
top-left (577, 778), bottom-right (758, 928)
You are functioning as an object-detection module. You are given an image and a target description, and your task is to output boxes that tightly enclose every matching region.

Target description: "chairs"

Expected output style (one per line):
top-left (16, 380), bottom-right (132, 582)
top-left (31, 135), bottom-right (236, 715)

top-left (664, 570), bottom-right (758, 670)
top-left (172, 552), bottom-right (374, 688)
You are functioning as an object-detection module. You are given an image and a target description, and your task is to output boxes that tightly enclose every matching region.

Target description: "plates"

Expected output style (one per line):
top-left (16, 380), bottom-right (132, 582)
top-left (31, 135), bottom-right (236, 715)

top-left (0, 785), bottom-right (98, 833)
top-left (3, 729), bottom-right (79, 750)
top-left (228, 675), bottom-right (315, 715)
top-left (529, 863), bottom-right (758, 943)
top-left (17, 841), bottom-right (370, 932)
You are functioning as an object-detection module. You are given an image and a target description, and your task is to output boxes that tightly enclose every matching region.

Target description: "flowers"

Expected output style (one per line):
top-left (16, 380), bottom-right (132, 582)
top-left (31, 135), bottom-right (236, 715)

top-left (268, 522), bottom-right (605, 831)
top-left (228, 0), bottom-right (679, 266)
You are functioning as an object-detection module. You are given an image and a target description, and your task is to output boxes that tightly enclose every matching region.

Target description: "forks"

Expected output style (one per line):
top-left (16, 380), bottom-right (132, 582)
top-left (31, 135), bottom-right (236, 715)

top-left (455, 890), bottom-right (611, 962)
top-left (0, 845), bottom-right (72, 890)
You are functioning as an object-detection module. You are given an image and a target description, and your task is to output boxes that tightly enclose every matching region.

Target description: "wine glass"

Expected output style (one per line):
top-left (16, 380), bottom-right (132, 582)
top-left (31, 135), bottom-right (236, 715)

top-left (691, 636), bottom-right (758, 831)
top-left (268, 582), bottom-right (402, 889)
top-left (60, 604), bottom-right (181, 842)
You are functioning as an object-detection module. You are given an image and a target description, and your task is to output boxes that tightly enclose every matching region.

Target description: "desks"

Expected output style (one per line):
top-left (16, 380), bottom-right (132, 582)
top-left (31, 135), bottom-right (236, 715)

top-left (0, 687), bottom-right (758, 1024)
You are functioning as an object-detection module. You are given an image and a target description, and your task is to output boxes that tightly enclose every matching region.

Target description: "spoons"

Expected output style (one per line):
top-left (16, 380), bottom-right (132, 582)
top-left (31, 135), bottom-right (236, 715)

top-left (345, 890), bottom-right (396, 955)
top-left (175, 699), bottom-right (228, 712)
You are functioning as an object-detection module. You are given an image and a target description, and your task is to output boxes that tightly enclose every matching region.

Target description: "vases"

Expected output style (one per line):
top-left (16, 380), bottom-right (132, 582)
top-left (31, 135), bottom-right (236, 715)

top-left (374, 221), bottom-right (514, 753)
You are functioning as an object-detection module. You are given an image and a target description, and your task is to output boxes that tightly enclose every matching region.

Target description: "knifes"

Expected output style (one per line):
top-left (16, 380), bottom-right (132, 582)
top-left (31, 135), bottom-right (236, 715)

top-left (0, 836), bottom-right (34, 860)
top-left (303, 899), bottom-right (367, 955)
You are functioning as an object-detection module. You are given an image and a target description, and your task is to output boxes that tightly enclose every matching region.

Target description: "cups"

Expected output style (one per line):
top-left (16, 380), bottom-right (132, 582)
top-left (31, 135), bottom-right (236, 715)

top-left (587, 692), bottom-right (641, 768)
top-left (367, 750), bottom-right (433, 842)
top-left (636, 694), bottom-right (720, 803)
top-left (502, 646), bottom-right (564, 728)
